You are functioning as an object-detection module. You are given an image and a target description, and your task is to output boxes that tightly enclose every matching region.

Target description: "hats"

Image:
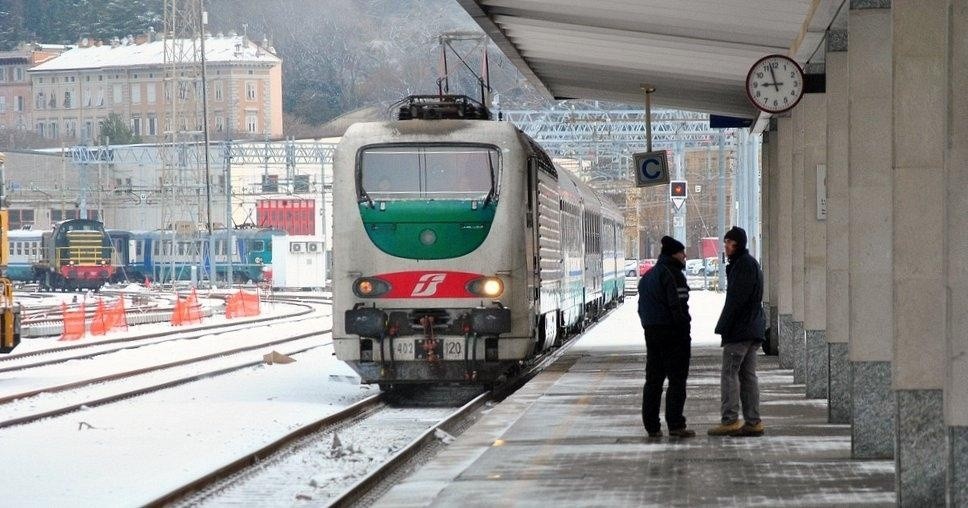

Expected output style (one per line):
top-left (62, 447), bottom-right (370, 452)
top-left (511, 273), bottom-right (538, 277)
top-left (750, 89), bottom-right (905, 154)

top-left (661, 236), bottom-right (684, 256)
top-left (724, 227), bottom-right (747, 247)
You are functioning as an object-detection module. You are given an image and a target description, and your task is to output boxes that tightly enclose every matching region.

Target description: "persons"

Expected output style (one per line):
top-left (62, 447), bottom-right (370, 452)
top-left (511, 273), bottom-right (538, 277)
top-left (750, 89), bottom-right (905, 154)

top-left (638, 235), bottom-right (696, 438)
top-left (706, 225), bottom-right (766, 438)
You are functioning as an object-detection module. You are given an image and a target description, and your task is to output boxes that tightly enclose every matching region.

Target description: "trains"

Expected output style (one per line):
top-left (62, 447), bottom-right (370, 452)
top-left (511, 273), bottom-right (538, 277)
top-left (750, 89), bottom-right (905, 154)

top-left (6, 217), bottom-right (290, 293)
top-left (0, 151), bottom-right (22, 355)
top-left (31, 217), bottom-right (117, 292)
top-left (332, 94), bottom-right (625, 403)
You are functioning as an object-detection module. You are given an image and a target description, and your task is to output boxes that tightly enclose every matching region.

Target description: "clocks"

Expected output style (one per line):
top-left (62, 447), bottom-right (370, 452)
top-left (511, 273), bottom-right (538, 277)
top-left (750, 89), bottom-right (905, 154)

top-left (744, 52), bottom-right (807, 113)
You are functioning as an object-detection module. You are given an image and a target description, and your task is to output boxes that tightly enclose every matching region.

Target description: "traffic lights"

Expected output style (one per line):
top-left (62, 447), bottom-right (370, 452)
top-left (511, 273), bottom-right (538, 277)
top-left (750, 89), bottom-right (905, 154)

top-left (670, 180), bottom-right (688, 199)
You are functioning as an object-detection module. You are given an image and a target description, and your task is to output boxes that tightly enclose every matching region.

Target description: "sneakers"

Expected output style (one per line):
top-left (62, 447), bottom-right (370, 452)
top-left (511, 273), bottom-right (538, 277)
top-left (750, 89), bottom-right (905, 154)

top-left (730, 423), bottom-right (764, 436)
top-left (669, 428), bottom-right (695, 438)
top-left (708, 419), bottom-right (740, 435)
top-left (650, 430), bottom-right (663, 436)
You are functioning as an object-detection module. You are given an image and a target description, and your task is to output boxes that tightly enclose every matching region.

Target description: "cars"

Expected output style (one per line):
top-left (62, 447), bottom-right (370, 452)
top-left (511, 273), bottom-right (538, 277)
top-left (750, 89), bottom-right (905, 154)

top-left (624, 256), bottom-right (729, 277)
